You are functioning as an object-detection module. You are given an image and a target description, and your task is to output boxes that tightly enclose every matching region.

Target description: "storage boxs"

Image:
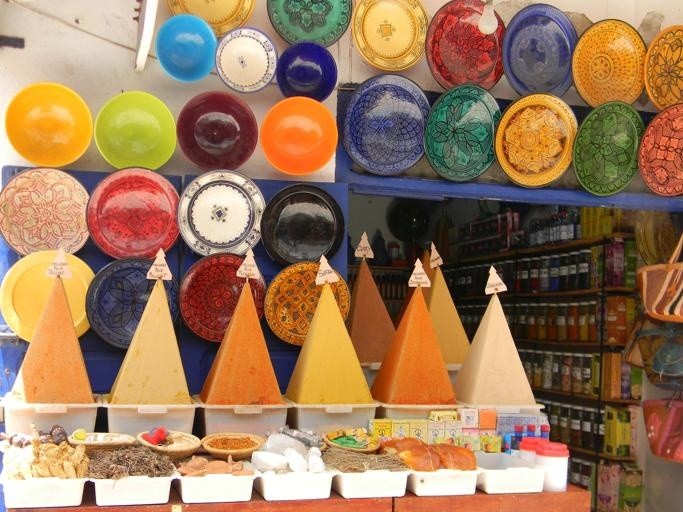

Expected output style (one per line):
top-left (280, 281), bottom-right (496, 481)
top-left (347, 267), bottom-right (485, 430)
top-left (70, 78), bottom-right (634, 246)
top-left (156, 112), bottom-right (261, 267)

top-left (253, 470), bottom-right (338, 501)
top-left (375, 400), bottom-right (464, 420)
top-left (406, 469), bottom-right (482, 496)
top-left (175, 460), bottom-right (259, 504)
top-left (284, 399), bottom-right (382, 434)
top-left (465, 404), bottom-right (545, 415)
top-left (331, 468), bottom-right (411, 499)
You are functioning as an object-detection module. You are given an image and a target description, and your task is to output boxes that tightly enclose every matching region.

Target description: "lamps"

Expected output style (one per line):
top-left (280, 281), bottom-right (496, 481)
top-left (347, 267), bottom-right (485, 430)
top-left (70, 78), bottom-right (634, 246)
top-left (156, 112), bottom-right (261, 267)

top-left (478, 0), bottom-right (498, 34)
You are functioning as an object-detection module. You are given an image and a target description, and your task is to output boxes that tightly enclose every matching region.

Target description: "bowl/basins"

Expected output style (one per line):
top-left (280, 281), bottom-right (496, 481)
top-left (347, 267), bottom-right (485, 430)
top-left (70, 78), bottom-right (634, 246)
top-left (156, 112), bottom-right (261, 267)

top-left (372, 397), bottom-right (467, 421)
top-left (444, 363), bottom-right (462, 388)
top-left (455, 398), bottom-right (545, 418)
top-left (329, 463), bottom-right (412, 499)
top-left (243, 458), bottom-right (337, 500)
top-left (359, 361), bottom-right (383, 390)
top-left (169, 457), bottom-right (260, 503)
top-left (281, 393), bottom-right (381, 436)
top-left (407, 463), bottom-right (482, 497)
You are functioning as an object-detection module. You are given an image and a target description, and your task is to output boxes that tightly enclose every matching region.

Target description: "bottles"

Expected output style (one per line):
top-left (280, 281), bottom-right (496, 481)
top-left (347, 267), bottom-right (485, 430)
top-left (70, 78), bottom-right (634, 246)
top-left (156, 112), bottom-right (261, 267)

top-left (518, 349), bottom-right (533, 385)
top-left (580, 250), bottom-right (591, 289)
top-left (519, 303), bottom-right (528, 339)
top-left (530, 206), bottom-right (581, 246)
top-left (581, 463), bottom-right (594, 489)
top-left (374, 273), bottom-right (414, 299)
top-left (570, 458), bottom-right (582, 485)
top-left (540, 257), bottom-right (552, 292)
top-left (572, 353), bottom-right (583, 397)
top-left (515, 261), bottom-right (520, 291)
top-left (548, 403), bottom-right (561, 440)
top-left (520, 259), bottom-right (530, 292)
top-left (529, 303), bottom-right (538, 339)
top-left (568, 303), bottom-right (579, 342)
top-left (445, 265), bottom-right (511, 299)
top-left (558, 303), bottom-right (566, 341)
top-left (536, 398), bottom-right (549, 414)
top-left (527, 425), bottom-right (537, 441)
top-left (454, 305), bottom-right (488, 340)
top-left (540, 425), bottom-right (550, 441)
top-left (571, 253), bottom-right (579, 291)
top-left (561, 255), bottom-right (571, 291)
top-left (538, 303), bottom-right (547, 340)
top-left (550, 255), bottom-right (560, 291)
top-left (561, 404), bottom-right (571, 444)
top-left (562, 353), bottom-right (570, 392)
top-left (583, 354), bottom-right (592, 394)
top-left (542, 352), bottom-right (553, 389)
top-left (389, 243), bottom-right (400, 264)
top-left (513, 426), bottom-right (523, 448)
top-left (502, 303), bottom-right (517, 337)
top-left (530, 259), bottom-right (540, 293)
top-left (548, 304), bottom-right (557, 340)
top-left (599, 411), bottom-right (607, 450)
top-left (553, 353), bottom-right (563, 391)
top-left (583, 409), bottom-right (597, 450)
top-left (533, 351), bottom-right (542, 389)
top-left (580, 303), bottom-right (588, 341)
top-left (571, 407), bottom-right (583, 447)
top-left (588, 302), bottom-right (598, 342)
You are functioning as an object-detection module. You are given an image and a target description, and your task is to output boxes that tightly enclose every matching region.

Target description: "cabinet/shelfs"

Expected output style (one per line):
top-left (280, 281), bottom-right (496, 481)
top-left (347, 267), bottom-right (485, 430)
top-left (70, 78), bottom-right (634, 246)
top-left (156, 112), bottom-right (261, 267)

top-left (440, 233), bottom-right (640, 512)
top-left (4, 484), bottom-right (591, 512)
top-left (345, 266), bottom-right (413, 324)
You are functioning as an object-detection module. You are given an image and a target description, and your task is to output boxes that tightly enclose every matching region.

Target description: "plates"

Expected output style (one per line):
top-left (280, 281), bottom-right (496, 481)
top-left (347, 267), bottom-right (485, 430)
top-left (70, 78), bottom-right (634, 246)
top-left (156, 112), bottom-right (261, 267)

top-left (424, 0), bottom-right (507, 93)
top-left (258, 94), bottom-right (339, 177)
top-left (4, 80), bottom-right (96, 167)
top-left (177, 88), bottom-right (259, 172)
top-left (350, 0), bottom-right (430, 72)
top-left (85, 166), bottom-right (182, 261)
top-left (423, 82), bottom-right (505, 183)
top-left (259, 182), bottom-right (346, 266)
top-left (571, 18), bottom-right (648, 110)
top-left (155, 12), bottom-right (217, 82)
top-left (265, 1), bottom-right (355, 49)
top-left (274, 41), bottom-right (338, 103)
top-left (572, 102), bottom-right (646, 197)
top-left (163, 2), bottom-right (255, 39)
top-left (84, 256), bottom-right (181, 351)
top-left (494, 93), bottom-right (579, 190)
top-left (342, 73), bottom-right (432, 175)
top-left (177, 251), bottom-right (268, 344)
top-left (502, 4), bottom-right (580, 99)
top-left (176, 168), bottom-right (267, 256)
top-left (643, 25), bottom-right (682, 113)
top-left (638, 103), bottom-right (682, 199)
top-left (263, 260), bottom-right (352, 348)
top-left (215, 28), bottom-right (277, 95)
top-left (2, 167), bottom-right (91, 256)
top-left (0, 249), bottom-right (97, 344)
top-left (93, 90), bottom-right (177, 172)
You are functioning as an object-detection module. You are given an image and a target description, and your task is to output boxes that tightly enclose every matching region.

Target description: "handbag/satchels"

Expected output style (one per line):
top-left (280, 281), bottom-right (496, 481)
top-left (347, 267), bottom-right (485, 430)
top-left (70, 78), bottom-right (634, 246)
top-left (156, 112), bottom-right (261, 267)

top-left (638, 232), bottom-right (683, 322)
top-left (643, 392), bottom-right (683, 464)
top-left (637, 328), bottom-right (682, 390)
